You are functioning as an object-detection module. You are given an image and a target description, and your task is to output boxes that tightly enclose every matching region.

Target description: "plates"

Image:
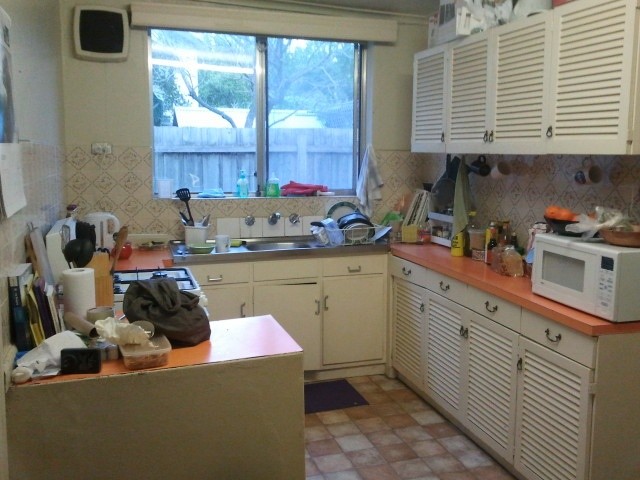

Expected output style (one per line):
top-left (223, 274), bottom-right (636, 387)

top-left (344, 223), bottom-right (364, 234)
top-left (327, 201), bottom-right (361, 219)
top-left (543, 216), bottom-right (600, 236)
top-left (345, 224), bottom-right (369, 240)
top-left (342, 224), bottom-right (351, 231)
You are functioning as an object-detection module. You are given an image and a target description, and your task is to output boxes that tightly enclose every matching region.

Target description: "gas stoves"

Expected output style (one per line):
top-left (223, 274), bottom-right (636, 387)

top-left (46, 217), bottom-right (209, 323)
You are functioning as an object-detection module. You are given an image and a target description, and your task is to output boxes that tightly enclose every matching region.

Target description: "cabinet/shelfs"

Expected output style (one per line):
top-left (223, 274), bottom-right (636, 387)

top-left (253, 253), bottom-right (388, 377)
top-left (410, 42), bottom-right (448, 153)
top-left (445, 9), bottom-right (553, 154)
top-left (553, 0), bottom-right (640, 155)
top-left (173, 261), bottom-right (250, 322)
top-left (5, 314), bottom-right (304, 480)
top-left (426, 268), bottom-right (522, 473)
top-left (513, 307), bottom-right (640, 479)
top-left (389, 253), bottom-right (426, 400)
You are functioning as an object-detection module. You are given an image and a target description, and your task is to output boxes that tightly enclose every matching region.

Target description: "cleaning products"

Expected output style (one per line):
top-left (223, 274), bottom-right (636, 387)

top-left (236, 170), bottom-right (249, 197)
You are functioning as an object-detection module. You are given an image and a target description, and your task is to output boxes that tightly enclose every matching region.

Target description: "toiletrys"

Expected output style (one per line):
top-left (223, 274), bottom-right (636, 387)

top-left (266, 172), bottom-right (280, 197)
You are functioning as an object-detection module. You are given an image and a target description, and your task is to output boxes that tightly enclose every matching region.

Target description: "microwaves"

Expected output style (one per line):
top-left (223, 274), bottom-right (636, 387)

top-left (532, 231), bottom-right (640, 323)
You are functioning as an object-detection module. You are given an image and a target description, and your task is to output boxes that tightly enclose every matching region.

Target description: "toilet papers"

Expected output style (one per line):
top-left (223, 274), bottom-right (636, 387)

top-left (62, 268), bottom-right (96, 330)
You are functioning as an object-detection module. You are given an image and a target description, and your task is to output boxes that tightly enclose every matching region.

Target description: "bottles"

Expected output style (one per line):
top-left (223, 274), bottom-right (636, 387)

top-left (464, 211), bottom-right (480, 257)
top-left (87, 305), bottom-right (114, 324)
top-left (423, 183), bottom-right (433, 192)
top-left (447, 208), bottom-right (452, 215)
top-left (511, 232), bottom-right (518, 250)
top-left (497, 224), bottom-right (507, 245)
top-left (486, 228), bottom-right (497, 264)
top-left (442, 226), bottom-right (449, 239)
top-left (485, 221), bottom-right (499, 263)
top-left (156, 178), bottom-right (175, 198)
top-left (503, 218), bottom-right (510, 245)
top-left (310, 226), bottom-right (318, 235)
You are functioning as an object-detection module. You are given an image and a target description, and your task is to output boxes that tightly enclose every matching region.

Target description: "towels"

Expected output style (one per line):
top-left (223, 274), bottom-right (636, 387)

top-left (280, 180), bottom-right (328, 196)
top-left (451, 156), bottom-right (472, 241)
top-left (356, 143), bottom-right (383, 220)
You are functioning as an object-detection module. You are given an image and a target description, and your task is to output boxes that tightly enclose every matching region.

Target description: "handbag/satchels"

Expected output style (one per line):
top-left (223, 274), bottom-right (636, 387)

top-left (123, 277), bottom-right (211, 345)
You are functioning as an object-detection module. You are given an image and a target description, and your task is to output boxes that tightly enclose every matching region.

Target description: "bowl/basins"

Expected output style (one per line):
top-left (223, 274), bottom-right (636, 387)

top-left (187, 243), bottom-right (215, 254)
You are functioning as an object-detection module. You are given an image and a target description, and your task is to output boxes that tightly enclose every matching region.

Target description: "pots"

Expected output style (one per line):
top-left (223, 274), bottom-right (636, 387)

top-left (310, 213), bottom-right (375, 238)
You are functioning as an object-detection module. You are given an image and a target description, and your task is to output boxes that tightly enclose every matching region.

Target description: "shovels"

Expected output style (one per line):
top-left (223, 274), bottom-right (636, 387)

top-left (175, 188), bottom-right (194, 226)
top-left (108, 227), bottom-right (129, 275)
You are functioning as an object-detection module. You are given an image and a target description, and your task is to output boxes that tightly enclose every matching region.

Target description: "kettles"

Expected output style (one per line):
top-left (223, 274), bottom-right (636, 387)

top-left (85, 212), bottom-right (121, 253)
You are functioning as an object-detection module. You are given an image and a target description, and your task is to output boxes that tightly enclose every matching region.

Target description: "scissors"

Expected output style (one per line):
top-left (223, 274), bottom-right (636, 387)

top-left (96, 248), bottom-right (110, 259)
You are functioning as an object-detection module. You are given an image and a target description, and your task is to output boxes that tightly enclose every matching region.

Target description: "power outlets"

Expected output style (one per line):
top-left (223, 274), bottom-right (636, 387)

top-left (91, 142), bottom-right (112, 155)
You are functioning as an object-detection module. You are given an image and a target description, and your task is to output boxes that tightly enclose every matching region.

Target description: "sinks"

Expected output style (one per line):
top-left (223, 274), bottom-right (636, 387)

top-left (243, 241), bottom-right (312, 252)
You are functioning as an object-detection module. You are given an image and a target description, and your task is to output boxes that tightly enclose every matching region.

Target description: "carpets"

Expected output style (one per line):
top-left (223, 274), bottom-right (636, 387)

top-left (304, 378), bottom-right (369, 414)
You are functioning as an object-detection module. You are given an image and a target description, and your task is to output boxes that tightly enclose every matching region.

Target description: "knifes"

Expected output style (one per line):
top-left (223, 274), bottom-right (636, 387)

top-left (179, 210), bottom-right (192, 226)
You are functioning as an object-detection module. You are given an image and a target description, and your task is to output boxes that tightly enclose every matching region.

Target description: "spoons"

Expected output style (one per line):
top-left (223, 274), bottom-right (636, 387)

top-left (65, 240), bottom-right (82, 269)
top-left (73, 239), bottom-right (95, 268)
top-left (110, 226), bottom-right (129, 276)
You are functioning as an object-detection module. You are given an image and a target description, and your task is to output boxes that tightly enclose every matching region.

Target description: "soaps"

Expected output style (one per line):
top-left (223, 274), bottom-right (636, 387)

top-left (231, 240), bottom-right (242, 247)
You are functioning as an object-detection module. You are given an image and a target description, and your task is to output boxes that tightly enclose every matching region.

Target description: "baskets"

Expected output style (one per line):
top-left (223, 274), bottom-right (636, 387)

top-left (403, 188), bottom-right (431, 226)
top-left (314, 223), bottom-right (381, 246)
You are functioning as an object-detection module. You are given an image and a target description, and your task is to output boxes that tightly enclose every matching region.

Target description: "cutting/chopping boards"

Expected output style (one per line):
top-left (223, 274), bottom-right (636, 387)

top-left (24, 227), bottom-right (55, 284)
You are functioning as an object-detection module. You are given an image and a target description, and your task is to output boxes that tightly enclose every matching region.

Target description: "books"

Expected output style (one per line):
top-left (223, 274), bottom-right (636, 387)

top-left (6, 263), bottom-right (33, 351)
top-left (21, 270), bottom-right (64, 347)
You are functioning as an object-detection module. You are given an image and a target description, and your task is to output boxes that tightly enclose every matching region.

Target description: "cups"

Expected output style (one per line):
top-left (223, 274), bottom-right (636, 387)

top-left (470, 155), bottom-right (490, 176)
top-left (490, 155), bottom-right (513, 179)
top-left (312, 227), bottom-right (329, 246)
top-left (571, 156), bottom-right (603, 187)
top-left (214, 235), bottom-right (231, 252)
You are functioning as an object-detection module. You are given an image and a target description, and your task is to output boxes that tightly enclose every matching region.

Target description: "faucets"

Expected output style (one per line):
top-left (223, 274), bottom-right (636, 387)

top-left (268, 212), bottom-right (280, 225)
